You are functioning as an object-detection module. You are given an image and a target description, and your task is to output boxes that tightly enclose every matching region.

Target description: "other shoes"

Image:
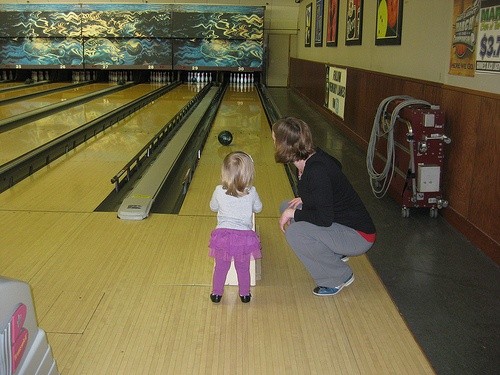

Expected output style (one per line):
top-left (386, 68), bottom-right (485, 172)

top-left (210, 294), bottom-right (222, 302)
top-left (341, 256), bottom-right (346, 259)
top-left (240, 293), bottom-right (251, 303)
top-left (313, 273), bottom-right (354, 296)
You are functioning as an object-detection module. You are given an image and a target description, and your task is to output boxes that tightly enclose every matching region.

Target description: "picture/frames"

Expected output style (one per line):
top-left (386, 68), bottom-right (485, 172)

top-left (304, 2), bottom-right (312, 47)
top-left (345, 0), bottom-right (364, 45)
top-left (314, 0), bottom-right (324, 47)
top-left (375, 0), bottom-right (403, 45)
top-left (326, 0), bottom-right (339, 47)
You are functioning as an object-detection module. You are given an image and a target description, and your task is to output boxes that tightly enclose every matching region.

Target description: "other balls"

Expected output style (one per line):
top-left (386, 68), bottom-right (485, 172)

top-left (218, 131), bottom-right (233, 146)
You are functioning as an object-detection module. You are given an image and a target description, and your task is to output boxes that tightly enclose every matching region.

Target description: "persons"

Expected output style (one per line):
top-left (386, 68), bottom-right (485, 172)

top-left (271, 116), bottom-right (376, 296)
top-left (208, 151), bottom-right (263, 303)
